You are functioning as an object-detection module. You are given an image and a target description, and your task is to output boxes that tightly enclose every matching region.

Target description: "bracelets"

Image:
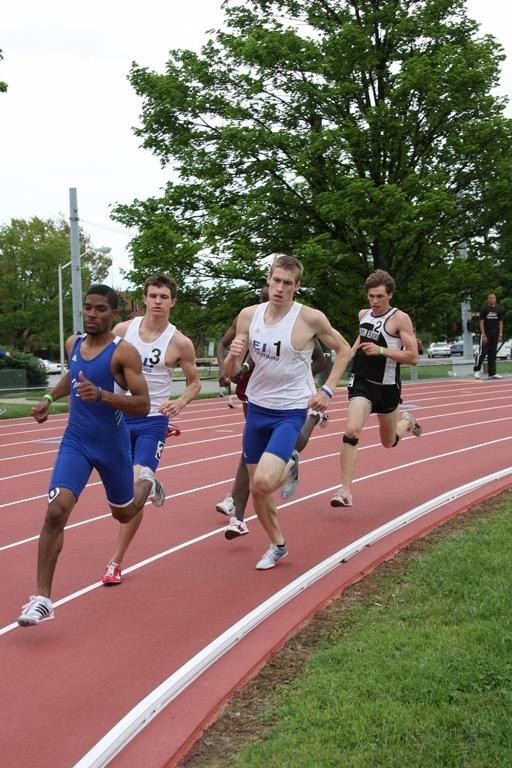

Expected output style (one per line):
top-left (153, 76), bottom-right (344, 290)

top-left (43, 394), bottom-right (54, 404)
top-left (498, 334), bottom-right (502, 336)
top-left (96, 386), bottom-right (102, 402)
top-left (243, 363), bottom-right (250, 371)
top-left (380, 345), bottom-right (384, 356)
top-left (321, 385), bottom-right (334, 398)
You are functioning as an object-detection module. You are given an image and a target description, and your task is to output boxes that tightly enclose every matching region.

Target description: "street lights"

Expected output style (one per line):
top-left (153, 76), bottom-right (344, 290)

top-left (56, 246), bottom-right (112, 378)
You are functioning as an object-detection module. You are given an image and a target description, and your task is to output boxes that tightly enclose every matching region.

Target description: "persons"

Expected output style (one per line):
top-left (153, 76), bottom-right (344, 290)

top-left (224, 283), bottom-right (329, 539)
top-left (222, 255), bottom-right (353, 572)
top-left (215, 312), bottom-right (296, 516)
top-left (331, 270), bottom-right (423, 508)
top-left (102, 275), bottom-right (201, 585)
top-left (473, 292), bottom-right (504, 379)
top-left (18, 283), bottom-right (164, 627)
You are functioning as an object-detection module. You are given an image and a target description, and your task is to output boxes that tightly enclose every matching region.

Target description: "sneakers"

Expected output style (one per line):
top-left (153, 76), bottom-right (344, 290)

top-left (101, 558), bottom-right (122, 585)
top-left (137, 464), bottom-right (167, 509)
top-left (252, 540), bottom-right (289, 570)
top-left (16, 595), bottom-right (56, 627)
top-left (329, 488), bottom-right (353, 508)
top-left (223, 516), bottom-right (249, 540)
top-left (165, 423), bottom-right (181, 438)
top-left (401, 410), bottom-right (423, 438)
top-left (216, 495), bottom-right (238, 518)
top-left (474, 370), bottom-right (504, 380)
top-left (276, 407), bottom-right (331, 499)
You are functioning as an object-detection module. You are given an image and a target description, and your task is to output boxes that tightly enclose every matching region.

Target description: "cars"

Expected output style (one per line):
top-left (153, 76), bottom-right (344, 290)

top-left (37, 357), bottom-right (69, 374)
top-left (415, 334), bottom-right (512, 362)
